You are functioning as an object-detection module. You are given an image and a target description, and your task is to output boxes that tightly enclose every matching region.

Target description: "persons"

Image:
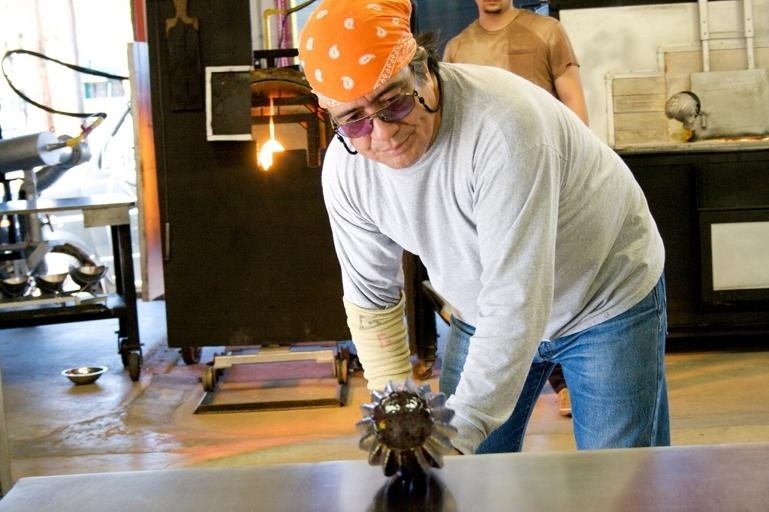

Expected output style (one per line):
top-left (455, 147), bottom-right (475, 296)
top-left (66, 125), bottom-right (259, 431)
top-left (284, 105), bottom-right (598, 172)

top-left (296, 0), bottom-right (673, 456)
top-left (440, 0), bottom-right (589, 129)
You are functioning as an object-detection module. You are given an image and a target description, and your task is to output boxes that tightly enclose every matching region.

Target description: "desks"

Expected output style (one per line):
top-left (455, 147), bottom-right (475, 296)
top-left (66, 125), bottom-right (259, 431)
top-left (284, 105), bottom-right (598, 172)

top-left (0, 442), bottom-right (769, 512)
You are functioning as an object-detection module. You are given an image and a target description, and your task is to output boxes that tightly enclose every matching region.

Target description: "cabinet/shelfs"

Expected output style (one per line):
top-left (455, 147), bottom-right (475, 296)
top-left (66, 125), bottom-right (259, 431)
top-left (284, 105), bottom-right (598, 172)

top-left (0, 190), bottom-right (143, 382)
top-left (607, 139), bottom-right (769, 353)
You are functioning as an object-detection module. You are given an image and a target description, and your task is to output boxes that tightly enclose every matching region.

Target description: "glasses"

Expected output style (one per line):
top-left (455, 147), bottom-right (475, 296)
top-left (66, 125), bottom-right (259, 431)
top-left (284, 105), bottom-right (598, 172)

top-left (330, 64), bottom-right (416, 138)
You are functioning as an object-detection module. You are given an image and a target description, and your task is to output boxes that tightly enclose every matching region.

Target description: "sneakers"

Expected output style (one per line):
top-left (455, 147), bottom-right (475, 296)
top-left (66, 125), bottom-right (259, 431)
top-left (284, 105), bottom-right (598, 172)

top-left (556, 391), bottom-right (572, 417)
top-left (415, 359), bottom-right (435, 378)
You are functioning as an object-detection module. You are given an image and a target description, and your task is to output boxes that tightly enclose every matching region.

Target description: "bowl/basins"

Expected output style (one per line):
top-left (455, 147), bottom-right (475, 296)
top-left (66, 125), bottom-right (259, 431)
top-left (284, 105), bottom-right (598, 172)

top-left (60, 366), bottom-right (107, 385)
